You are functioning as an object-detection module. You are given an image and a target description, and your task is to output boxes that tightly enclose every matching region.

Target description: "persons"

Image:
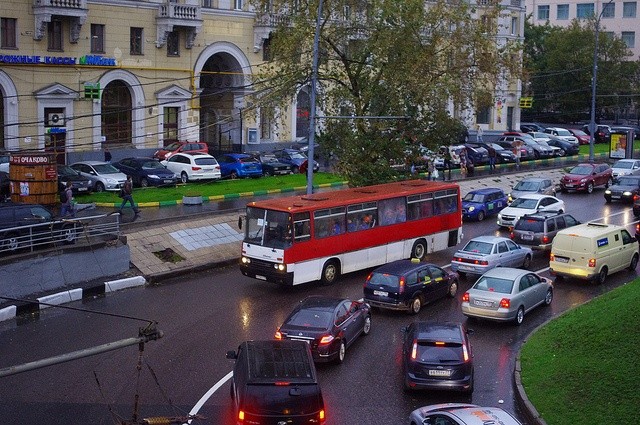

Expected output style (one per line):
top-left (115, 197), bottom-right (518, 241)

top-left (448, 197), bottom-right (456, 212)
top-left (458, 148), bottom-right (468, 174)
top-left (61, 182), bottom-right (76, 219)
top-left (488, 145), bottom-right (496, 171)
top-left (443, 147), bottom-right (453, 175)
top-left (428, 155), bottom-right (438, 182)
top-left (396, 203), bottom-right (406, 222)
top-left (435, 204), bottom-right (440, 215)
top-left (331, 218), bottom-right (341, 235)
top-left (513, 142), bottom-right (521, 168)
top-left (358, 216), bottom-right (371, 231)
top-left (383, 207), bottom-right (396, 225)
top-left (348, 219), bottom-right (356, 231)
top-left (117, 175), bottom-right (141, 216)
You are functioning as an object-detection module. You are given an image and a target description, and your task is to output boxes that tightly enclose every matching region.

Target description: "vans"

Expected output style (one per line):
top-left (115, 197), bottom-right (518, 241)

top-left (584, 124), bottom-right (611, 142)
top-left (549, 221), bottom-right (639, 284)
top-left (520, 122), bottom-right (545, 133)
top-left (560, 163), bottom-right (613, 193)
top-left (611, 158), bottom-right (640, 178)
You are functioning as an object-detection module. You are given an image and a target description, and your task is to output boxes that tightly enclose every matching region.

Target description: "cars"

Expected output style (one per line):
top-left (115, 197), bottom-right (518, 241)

top-left (530, 131), bottom-right (555, 144)
top-left (568, 128), bottom-right (595, 144)
top-left (262, 158), bottom-right (292, 175)
top-left (409, 402), bottom-right (523, 424)
top-left (290, 137), bottom-right (319, 151)
top-left (215, 153), bottom-right (262, 178)
top-left (58, 166), bottom-right (93, 193)
top-left (496, 135), bottom-right (554, 157)
top-left (546, 137), bottom-right (579, 156)
top-left (274, 296), bottom-right (371, 364)
top-left (501, 141), bottom-right (534, 160)
top-left (112, 156), bottom-right (176, 186)
top-left (68, 160), bottom-right (133, 191)
top-left (269, 148), bottom-right (319, 173)
top-left (604, 175), bottom-right (640, 201)
top-left (244, 150), bottom-right (278, 163)
top-left (461, 266), bottom-right (553, 325)
top-left (451, 235), bottom-right (533, 276)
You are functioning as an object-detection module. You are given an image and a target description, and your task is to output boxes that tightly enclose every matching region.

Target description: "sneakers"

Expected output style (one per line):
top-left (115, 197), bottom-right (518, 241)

top-left (118, 210), bottom-right (124, 215)
top-left (135, 210), bottom-right (140, 214)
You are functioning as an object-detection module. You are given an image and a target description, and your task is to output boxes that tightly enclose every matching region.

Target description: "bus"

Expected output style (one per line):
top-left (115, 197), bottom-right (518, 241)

top-left (238, 178), bottom-right (463, 286)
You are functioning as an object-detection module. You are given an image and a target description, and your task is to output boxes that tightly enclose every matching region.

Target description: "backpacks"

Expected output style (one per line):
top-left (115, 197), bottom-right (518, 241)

top-left (60, 188), bottom-right (70, 203)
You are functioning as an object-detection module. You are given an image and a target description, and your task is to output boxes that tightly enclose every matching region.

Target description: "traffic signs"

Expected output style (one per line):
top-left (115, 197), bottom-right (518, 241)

top-left (519, 96), bottom-right (533, 107)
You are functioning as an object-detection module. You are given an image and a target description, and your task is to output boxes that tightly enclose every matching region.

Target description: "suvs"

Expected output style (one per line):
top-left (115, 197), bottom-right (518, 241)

top-left (464, 143), bottom-right (489, 164)
top-left (546, 126), bottom-right (579, 144)
top-left (226, 339), bottom-right (326, 424)
top-left (507, 175), bottom-right (556, 206)
top-left (0, 203), bottom-right (83, 252)
top-left (510, 211), bottom-right (582, 248)
top-left (484, 142), bottom-right (515, 162)
top-left (160, 151), bottom-right (221, 182)
top-left (497, 193), bottom-right (565, 228)
top-left (363, 257), bottom-right (460, 313)
top-left (400, 320), bottom-right (475, 394)
top-left (154, 139), bottom-right (208, 159)
top-left (462, 186), bottom-right (508, 220)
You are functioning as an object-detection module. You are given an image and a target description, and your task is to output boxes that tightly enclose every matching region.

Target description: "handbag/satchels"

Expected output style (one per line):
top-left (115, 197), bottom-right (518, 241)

top-left (513, 149), bottom-right (516, 154)
top-left (118, 189), bottom-right (124, 198)
